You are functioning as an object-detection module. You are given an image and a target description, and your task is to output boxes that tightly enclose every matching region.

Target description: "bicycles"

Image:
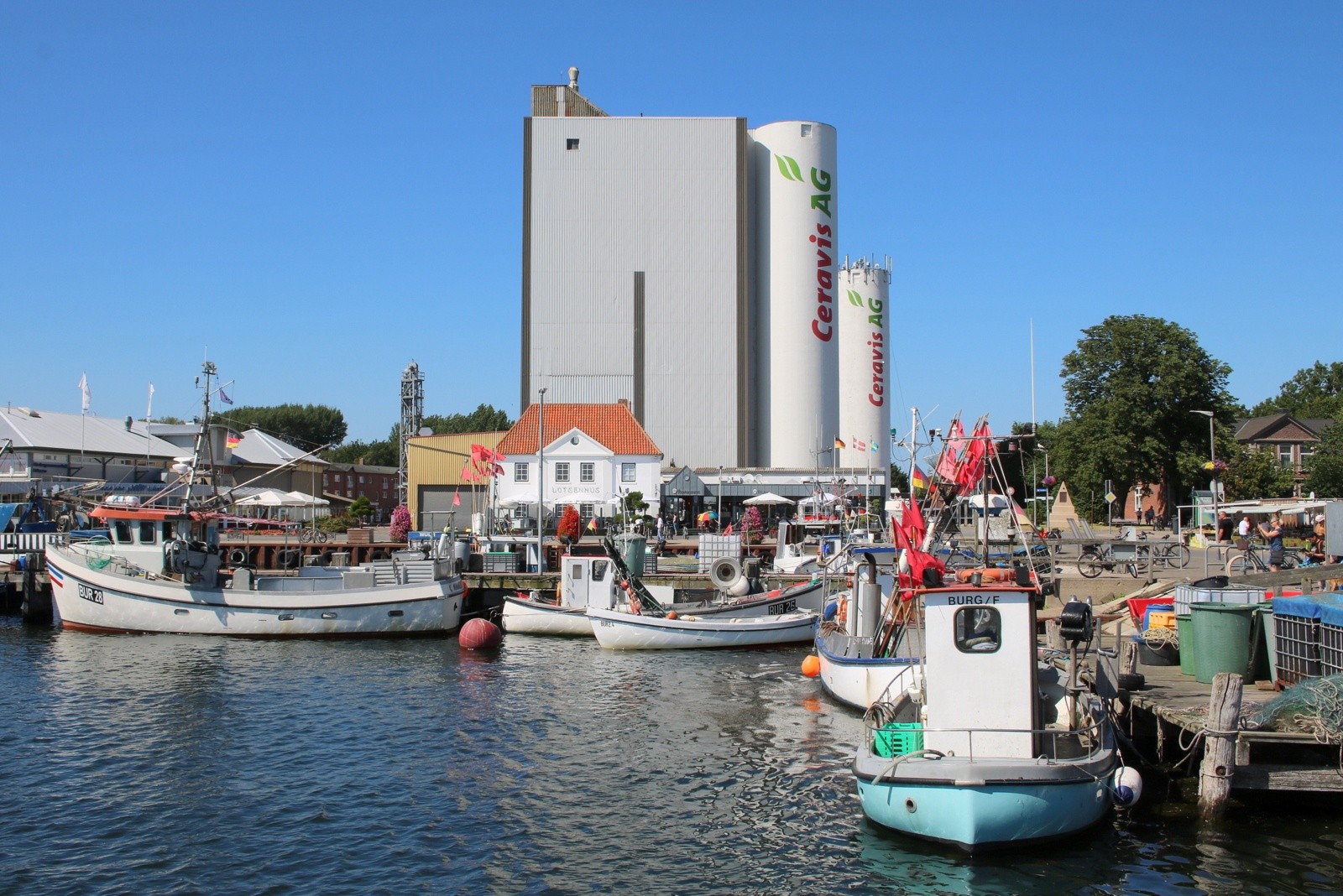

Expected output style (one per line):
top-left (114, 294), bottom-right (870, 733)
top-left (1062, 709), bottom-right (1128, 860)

top-left (1075, 514), bottom-right (1192, 580)
top-left (1224, 532), bottom-right (1306, 580)
top-left (300, 525), bottom-right (328, 543)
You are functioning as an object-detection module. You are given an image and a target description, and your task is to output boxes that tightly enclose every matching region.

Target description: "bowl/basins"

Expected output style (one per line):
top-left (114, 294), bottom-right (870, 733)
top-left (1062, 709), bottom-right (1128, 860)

top-left (1137, 640), bottom-right (1179, 666)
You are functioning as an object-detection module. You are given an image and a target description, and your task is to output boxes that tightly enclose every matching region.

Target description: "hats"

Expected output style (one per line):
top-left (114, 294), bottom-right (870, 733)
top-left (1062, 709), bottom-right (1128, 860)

top-left (1315, 515), bottom-right (1325, 522)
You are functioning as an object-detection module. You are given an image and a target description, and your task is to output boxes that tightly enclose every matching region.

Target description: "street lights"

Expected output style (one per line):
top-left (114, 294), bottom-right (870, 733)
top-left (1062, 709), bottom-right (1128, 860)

top-left (1187, 409), bottom-right (1219, 547)
top-left (718, 464), bottom-right (723, 537)
top-left (537, 386), bottom-right (548, 576)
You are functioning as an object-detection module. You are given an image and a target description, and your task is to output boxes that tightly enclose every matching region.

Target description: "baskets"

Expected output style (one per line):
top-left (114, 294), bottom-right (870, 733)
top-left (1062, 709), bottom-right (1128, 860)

top-left (1236, 539), bottom-right (1249, 551)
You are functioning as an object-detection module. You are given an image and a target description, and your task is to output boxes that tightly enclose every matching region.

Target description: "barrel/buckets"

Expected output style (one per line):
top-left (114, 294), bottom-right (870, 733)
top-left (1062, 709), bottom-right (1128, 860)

top-left (1189, 602), bottom-right (1259, 683)
top-left (1126, 597), bottom-right (1175, 632)
top-left (1191, 575), bottom-right (1229, 588)
top-left (1176, 614), bottom-right (1194, 675)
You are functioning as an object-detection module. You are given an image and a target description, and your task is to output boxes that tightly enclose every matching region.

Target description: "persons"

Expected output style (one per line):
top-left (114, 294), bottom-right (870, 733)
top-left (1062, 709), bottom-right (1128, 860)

top-left (1296, 515), bottom-right (1335, 591)
top-left (710, 520), bottom-right (716, 533)
top-left (1136, 506), bottom-right (1154, 525)
top-left (495, 513), bottom-right (512, 536)
top-left (673, 513), bottom-right (680, 536)
top-left (443, 523), bottom-right (452, 533)
top-left (1216, 511), bottom-right (1285, 572)
top-left (656, 512), bottom-right (663, 542)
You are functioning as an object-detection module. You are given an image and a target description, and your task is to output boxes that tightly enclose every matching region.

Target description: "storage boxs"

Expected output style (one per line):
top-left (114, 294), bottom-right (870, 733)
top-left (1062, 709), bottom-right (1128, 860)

top-left (874, 723), bottom-right (924, 759)
top-left (483, 552), bottom-right (521, 573)
top-left (1148, 613), bottom-right (1176, 633)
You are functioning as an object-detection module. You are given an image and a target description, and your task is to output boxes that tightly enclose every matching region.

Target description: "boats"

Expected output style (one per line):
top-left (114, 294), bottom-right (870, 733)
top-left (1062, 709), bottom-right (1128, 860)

top-left (494, 533), bottom-right (827, 638)
top-left (799, 424), bottom-right (1062, 719)
top-left (846, 418), bottom-right (1146, 857)
top-left (584, 605), bottom-right (829, 652)
top-left (41, 361), bottom-right (470, 638)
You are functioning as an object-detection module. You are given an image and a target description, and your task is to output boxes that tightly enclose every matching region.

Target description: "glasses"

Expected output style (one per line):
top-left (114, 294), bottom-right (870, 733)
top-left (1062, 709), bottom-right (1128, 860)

top-left (1270, 520), bottom-right (1276, 524)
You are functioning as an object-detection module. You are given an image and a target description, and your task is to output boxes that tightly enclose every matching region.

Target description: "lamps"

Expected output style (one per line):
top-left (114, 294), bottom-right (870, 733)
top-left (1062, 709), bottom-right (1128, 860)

top-left (971, 572), bottom-right (982, 588)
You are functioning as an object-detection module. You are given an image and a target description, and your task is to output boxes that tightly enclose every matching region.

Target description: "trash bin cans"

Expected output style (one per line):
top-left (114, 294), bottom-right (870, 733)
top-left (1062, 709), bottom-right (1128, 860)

top-left (1176, 613), bottom-right (1194, 675)
top-left (1189, 600), bottom-right (1260, 686)
top-left (454, 539), bottom-right (471, 571)
top-left (1259, 606), bottom-right (1291, 689)
top-left (613, 532), bottom-right (647, 578)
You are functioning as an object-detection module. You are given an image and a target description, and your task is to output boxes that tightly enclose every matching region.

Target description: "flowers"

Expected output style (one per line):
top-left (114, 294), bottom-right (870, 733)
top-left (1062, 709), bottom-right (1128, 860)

top-left (1041, 476), bottom-right (1057, 486)
top-left (741, 506), bottom-right (764, 543)
top-left (1199, 458), bottom-right (1229, 471)
top-left (389, 505), bottom-right (413, 543)
top-left (557, 504), bottom-right (584, 540)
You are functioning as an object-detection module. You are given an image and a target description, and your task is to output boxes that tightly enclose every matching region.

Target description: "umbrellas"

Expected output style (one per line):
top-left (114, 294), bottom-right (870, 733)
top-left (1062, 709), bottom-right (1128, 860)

top-left (234, 490), bottom-right (330, 523)
top-left (744, 492), bottom-right (851, 522)
top-left (698, 511), bottom-right (718, 522)
top-left (499, 493), bottom-right (661, 524)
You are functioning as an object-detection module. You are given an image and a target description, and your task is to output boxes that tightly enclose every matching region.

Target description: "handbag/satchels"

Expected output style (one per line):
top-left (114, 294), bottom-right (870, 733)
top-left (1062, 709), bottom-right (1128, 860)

top-left (1270, 550), bottom-right (1284, 564)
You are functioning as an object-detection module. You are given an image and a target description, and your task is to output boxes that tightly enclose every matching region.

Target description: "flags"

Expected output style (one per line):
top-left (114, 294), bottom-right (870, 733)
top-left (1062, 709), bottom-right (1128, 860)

top-left (219, 387), bottom-right (234, 405)
top-left (79, 373), bottom-right (91, 414)
top-left (451, 444), bottom-right (506, 506)
top-left (587, 517), bottom-right (597, 536)
top-left (147, 385), bottom-right (154, 424)
top-left (226, 429), bottom-right (245, 447)
top-left (890, 420), bottom-right (997, 603)
top-left (835, 437), bottom-right (880, 453)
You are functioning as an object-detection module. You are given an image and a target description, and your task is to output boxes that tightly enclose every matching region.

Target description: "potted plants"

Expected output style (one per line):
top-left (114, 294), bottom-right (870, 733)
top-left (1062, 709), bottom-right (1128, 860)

top-left (346, 497), bottom-right (376, 544)
top-left (769, 524), bottom-right (779, 538)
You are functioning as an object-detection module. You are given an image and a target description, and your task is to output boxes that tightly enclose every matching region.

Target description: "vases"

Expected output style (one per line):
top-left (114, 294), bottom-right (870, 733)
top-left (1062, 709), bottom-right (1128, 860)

top-left (744, 539), bottom-right (761, 544)
top-left (1202, 469), bottom-right (1223, 476)
top-left (560, 537), bottom-right (578, 544)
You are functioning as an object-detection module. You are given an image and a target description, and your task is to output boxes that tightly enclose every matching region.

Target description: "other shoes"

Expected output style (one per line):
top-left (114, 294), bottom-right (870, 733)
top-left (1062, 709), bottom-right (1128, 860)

top-left (1221, 566), bottom-right (1225, 571)
top-left (1318, 587), bottom-right (1323, 591)
